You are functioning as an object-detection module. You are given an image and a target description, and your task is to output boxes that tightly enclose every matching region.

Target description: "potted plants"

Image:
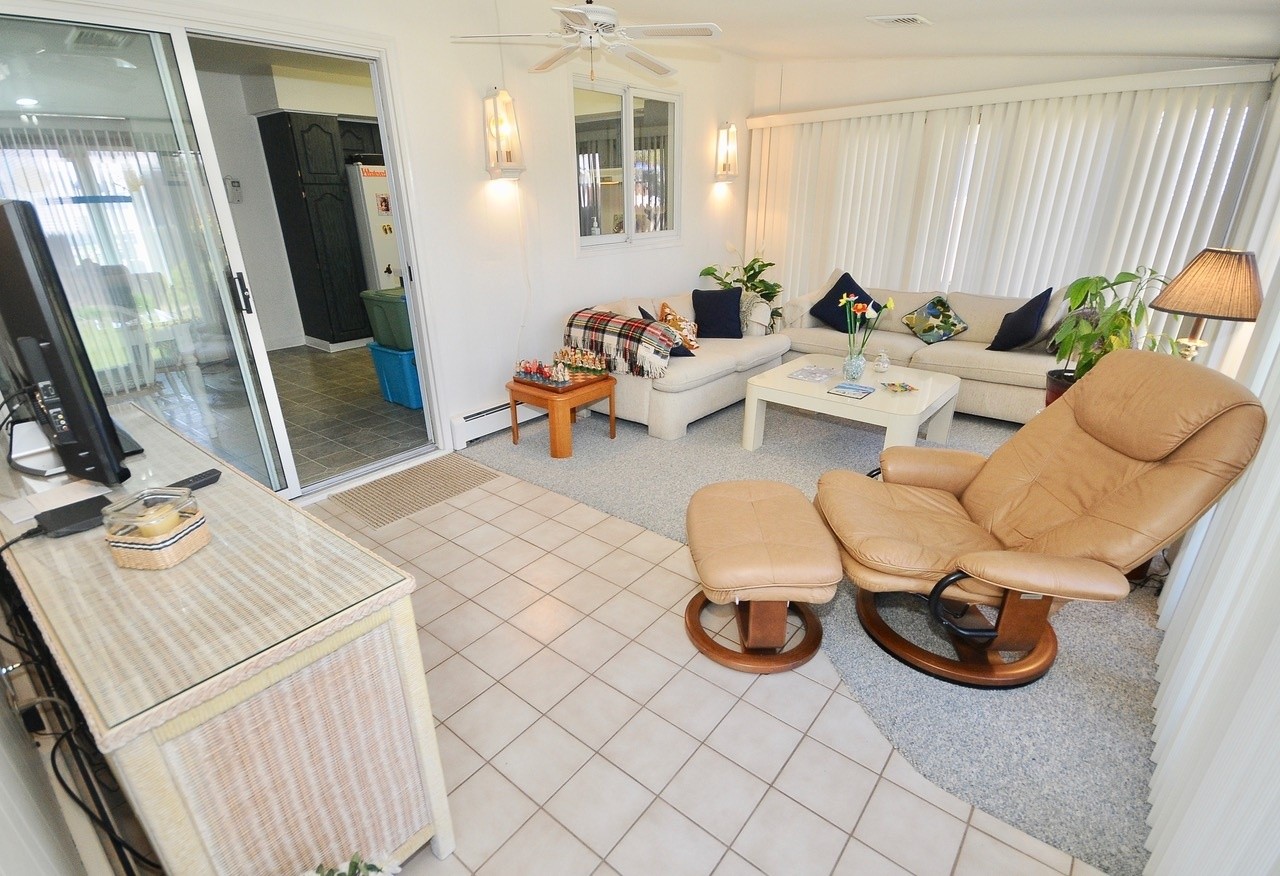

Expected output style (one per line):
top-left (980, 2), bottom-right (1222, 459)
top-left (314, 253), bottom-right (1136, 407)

top-left (1045, 266), bottom-right (1199, 408)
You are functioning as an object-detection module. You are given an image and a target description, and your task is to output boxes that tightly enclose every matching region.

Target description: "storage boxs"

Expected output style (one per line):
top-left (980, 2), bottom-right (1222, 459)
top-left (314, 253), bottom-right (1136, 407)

top-left (101, 488), bottom-right (210, 570)
top-left (357, 289), bottom-right (421, 410)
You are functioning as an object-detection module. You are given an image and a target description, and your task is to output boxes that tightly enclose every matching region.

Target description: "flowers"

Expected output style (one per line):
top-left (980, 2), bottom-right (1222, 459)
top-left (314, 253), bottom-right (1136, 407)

top-left (836, 292), bottom-right (896, 355)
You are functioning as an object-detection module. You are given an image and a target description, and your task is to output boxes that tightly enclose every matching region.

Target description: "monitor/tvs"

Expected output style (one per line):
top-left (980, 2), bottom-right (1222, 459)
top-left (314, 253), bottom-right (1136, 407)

top-left (0, 198), bottom-right (144, 489)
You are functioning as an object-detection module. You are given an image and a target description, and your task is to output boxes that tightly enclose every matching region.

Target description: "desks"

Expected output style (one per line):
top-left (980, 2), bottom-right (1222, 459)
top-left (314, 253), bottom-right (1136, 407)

top-left (2, 395), bottom-right (453, 876)
top-left (506, 373), bottom-right (619, 459)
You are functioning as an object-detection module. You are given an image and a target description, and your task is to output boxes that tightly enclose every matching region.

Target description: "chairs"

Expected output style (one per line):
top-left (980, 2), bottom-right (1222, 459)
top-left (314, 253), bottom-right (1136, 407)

top-left (682, 348), bottom-right (1267, 692)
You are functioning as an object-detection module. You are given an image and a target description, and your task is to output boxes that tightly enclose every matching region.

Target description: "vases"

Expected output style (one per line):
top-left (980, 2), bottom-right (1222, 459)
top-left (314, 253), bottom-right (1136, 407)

top-left (844, 345), bottom-right (866, 380)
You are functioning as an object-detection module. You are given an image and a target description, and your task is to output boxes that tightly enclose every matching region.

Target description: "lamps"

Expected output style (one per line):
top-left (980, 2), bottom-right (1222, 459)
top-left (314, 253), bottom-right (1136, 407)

top-left (483, 88), bottom-right (528, 174)
top-left (1149, 248), bottom-right (1264, 359)
top-left (582, 33), bottom-right (601, 80)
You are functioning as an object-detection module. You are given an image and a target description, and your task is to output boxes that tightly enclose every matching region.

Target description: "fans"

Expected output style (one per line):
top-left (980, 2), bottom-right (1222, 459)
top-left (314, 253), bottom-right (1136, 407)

top-left (450, 0), bottom-right (720, 78)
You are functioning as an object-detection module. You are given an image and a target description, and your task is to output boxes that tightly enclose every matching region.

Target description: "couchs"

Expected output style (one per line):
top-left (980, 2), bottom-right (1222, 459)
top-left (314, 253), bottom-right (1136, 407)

top-left (564, 285), bottom-right (791, 442)
top-left (784, 288), bottom-right (1097, 425)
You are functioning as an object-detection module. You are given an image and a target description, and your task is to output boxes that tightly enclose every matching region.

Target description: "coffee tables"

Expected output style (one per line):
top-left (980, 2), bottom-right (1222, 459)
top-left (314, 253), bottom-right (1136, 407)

top-left (744, 353), bottom-right (962, 482)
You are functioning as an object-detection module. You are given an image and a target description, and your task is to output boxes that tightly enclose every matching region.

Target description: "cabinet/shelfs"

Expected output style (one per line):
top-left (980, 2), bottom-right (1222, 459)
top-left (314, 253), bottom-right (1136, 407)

top-left (257, 111), bottom-right (387, 343)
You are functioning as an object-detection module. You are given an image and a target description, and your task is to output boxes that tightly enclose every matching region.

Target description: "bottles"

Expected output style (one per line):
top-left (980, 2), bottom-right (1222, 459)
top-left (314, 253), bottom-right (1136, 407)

top-left (874, 353), bottom-right (889, 372)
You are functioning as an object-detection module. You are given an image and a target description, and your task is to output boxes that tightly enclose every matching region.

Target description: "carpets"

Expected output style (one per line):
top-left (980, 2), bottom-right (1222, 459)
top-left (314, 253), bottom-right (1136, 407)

top-left (333, 452), bottom-right (499, 528)
top-left (463, 398), bottom-right (1157, 874)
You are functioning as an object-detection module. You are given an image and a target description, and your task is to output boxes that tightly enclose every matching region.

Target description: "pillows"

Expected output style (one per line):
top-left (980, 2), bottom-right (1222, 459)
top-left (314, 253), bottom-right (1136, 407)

top-left (810, 268), bottom-right (882, 333)
top-left (986, 283), bottom-right (1074, 351)
top-left (902, 296), bottom-right (967, 345)
top-left (692, 289), bottom-right (745, 340)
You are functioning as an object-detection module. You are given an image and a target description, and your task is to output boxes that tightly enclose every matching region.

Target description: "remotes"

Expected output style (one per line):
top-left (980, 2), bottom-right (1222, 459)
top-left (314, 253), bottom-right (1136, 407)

top-left (143, 468), bottom-right (222, 508)
